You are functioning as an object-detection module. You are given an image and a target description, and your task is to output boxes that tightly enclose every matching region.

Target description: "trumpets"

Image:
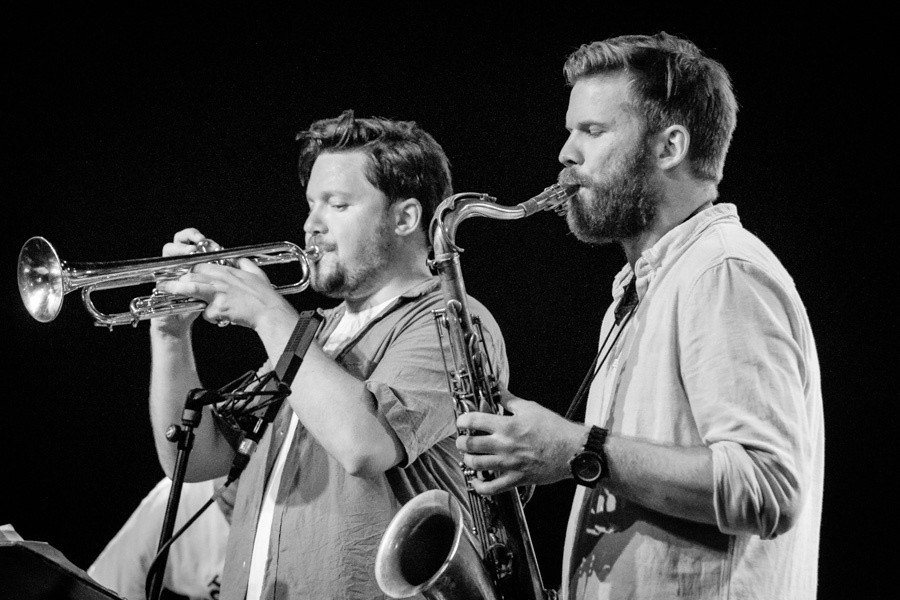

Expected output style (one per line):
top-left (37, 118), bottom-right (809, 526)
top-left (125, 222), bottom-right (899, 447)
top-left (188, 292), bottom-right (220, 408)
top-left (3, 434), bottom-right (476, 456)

top-left (18, 236), bottom-right (324, 334)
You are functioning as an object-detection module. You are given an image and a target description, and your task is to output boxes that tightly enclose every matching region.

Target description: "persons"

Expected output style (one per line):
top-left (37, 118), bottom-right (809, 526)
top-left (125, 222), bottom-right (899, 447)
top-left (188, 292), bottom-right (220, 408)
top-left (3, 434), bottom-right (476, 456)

top-left (75, 477), bottom-right (239, 600)
top-left (456, 31), bottom-right (825, 600)
top-left (147, 112), bottom-right (511, 600)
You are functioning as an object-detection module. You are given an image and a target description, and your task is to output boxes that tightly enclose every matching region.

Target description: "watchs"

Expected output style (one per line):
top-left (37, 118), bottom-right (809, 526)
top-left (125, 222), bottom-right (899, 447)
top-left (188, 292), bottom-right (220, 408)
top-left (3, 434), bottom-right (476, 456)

top-left (569, 426), bottom-right (609, 491)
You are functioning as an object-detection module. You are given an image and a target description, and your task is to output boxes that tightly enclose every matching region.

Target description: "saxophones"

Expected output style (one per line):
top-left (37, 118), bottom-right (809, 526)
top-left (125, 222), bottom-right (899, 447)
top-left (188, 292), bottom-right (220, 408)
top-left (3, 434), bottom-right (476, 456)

top-left (372, 180), bottom-right (580, 600)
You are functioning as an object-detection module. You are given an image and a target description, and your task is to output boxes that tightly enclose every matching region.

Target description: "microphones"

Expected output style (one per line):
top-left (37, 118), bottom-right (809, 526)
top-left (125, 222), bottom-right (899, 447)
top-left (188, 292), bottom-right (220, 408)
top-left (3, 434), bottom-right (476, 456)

top-left (224, 310), bottom-right (324, 481)
